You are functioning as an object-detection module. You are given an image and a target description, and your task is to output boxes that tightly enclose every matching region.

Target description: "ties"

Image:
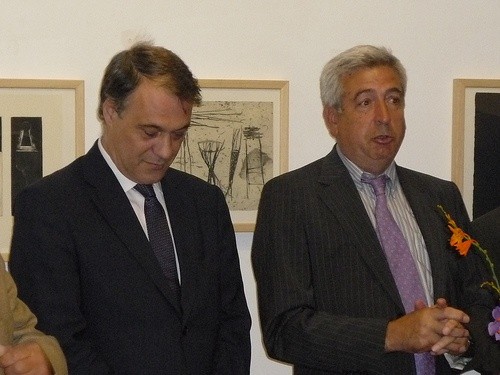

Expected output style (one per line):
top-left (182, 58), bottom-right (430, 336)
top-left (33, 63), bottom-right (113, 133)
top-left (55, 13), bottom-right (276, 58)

top-left (360, 173), bottom-right (434, 375)
top-left (133, 183), bottom-right (182, 309)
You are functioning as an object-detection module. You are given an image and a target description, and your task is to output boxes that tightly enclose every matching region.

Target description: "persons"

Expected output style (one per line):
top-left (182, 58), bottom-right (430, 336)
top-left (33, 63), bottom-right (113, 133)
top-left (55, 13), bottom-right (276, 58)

top-left (250, 45), bottom-right (500, 375)
top-left (472, 207), bottom-right (499, 286)
top-left (0, 253), bottom-right (68, 375)
top-left (5, 41), bottom-right (253, 375)
